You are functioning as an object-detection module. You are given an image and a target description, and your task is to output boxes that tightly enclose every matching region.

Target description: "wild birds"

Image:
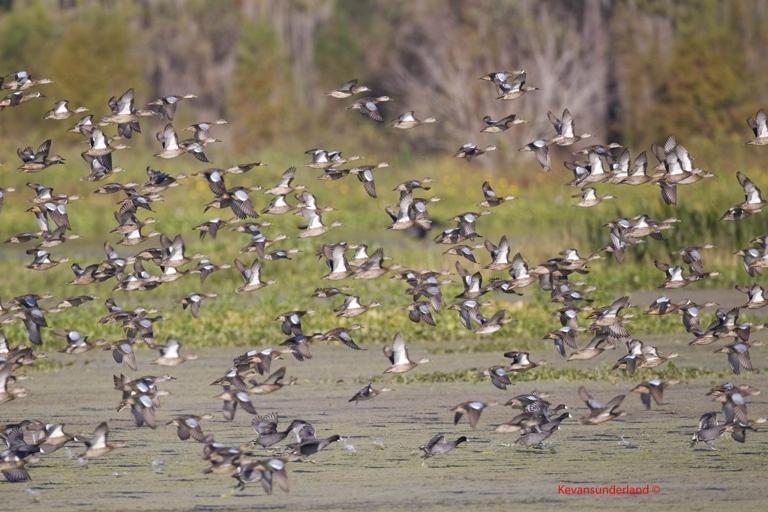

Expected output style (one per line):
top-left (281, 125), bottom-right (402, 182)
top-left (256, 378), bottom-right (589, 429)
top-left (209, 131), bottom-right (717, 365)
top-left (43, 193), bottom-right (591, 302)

top-left (0, 70), bottom-right (767, 497)
